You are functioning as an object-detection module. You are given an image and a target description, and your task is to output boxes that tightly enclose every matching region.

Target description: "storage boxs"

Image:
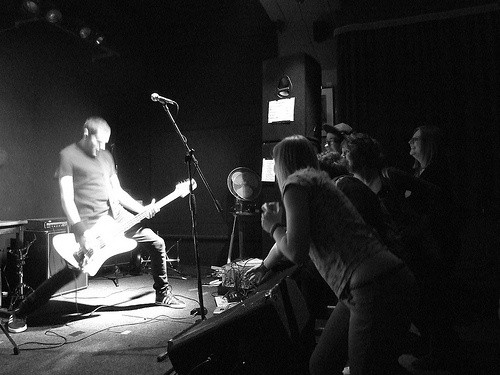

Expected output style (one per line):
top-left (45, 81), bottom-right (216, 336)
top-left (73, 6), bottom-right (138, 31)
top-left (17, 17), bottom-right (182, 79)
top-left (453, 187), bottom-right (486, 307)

top-left (23, 230), bottom-right (88, 298)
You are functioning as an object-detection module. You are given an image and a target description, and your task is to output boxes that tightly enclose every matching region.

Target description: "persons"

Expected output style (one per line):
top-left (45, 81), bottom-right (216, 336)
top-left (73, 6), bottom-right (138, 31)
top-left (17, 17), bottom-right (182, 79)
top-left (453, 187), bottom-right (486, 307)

top-left (8, 117), bottom-right (186, 332)
top-left (244, 121), bottom-right (461, 375)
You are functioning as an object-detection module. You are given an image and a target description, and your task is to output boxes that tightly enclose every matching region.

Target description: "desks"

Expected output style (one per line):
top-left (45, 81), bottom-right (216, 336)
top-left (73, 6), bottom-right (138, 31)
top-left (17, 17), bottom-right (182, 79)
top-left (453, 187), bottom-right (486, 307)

top-left (0, 220), bottom-right (28, 307)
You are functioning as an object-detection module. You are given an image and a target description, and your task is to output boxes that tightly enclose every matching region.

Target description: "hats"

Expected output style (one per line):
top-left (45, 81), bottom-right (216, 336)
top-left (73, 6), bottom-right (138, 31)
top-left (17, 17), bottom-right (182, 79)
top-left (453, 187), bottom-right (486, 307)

top-left (322, 123), bottom-right (353, 141)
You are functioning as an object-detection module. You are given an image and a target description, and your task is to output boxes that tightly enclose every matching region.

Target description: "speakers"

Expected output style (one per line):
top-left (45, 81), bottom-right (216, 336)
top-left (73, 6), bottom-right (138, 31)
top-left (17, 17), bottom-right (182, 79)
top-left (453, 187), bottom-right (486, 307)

top-left (22, 231), bottom-right (88, 297)
top-left (262, 52), bottom-right (322, 143)
top-left (167, 261), bottom-right (330, 375)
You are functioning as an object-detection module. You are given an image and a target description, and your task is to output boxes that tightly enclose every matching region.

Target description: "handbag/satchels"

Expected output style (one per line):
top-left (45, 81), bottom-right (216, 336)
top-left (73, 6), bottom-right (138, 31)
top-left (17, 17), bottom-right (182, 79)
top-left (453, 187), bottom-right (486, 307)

top-left (381, 167), bottom-right (436, 251)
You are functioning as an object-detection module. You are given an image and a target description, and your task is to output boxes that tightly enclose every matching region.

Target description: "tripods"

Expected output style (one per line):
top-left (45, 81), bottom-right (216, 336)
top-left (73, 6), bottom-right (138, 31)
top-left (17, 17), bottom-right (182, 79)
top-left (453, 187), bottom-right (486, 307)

top-left (89, 146), bottom-right (141, 288)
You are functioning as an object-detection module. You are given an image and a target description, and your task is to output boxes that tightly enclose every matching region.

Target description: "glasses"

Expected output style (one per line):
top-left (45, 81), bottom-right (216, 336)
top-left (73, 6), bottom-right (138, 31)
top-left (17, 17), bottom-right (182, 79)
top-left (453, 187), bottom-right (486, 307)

top-left (325, 141), bottom-right (339, 146)
top-left (411, 137), bottom-right (421, 142)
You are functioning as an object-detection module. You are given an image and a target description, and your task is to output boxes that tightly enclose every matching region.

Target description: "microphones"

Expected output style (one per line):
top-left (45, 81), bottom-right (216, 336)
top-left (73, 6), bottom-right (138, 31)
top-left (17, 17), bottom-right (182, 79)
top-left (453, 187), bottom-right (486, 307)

top-left (151, 93), bottom-right (178, 105)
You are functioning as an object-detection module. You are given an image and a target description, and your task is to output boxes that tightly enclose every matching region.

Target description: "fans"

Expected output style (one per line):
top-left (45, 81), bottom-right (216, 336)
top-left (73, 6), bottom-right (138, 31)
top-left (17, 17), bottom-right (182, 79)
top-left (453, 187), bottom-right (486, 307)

top-left (226, 167), bottom-right (261, 215)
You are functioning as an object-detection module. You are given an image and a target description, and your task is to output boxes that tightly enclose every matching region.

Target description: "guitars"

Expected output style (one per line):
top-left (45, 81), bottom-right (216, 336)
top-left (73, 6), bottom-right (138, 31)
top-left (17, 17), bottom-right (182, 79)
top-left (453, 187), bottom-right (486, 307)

top-left (52, 178), bottom-right (198, 278)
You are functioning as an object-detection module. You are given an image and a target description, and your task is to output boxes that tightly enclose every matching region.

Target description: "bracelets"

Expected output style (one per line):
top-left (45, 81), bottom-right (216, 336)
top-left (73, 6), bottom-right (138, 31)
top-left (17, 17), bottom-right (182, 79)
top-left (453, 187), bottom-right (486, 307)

top-left (271, 223), bottom-right (284, 237)
top-left (70, 221), bottom-right (87, 239)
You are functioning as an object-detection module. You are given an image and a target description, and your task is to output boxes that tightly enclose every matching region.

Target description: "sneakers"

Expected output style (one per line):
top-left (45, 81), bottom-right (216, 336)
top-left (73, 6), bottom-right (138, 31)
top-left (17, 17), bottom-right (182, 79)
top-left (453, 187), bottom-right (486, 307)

top-left (155, 285), bottom-right (186, 309)
top-left (7, 313), bottom-right (27, 332)
